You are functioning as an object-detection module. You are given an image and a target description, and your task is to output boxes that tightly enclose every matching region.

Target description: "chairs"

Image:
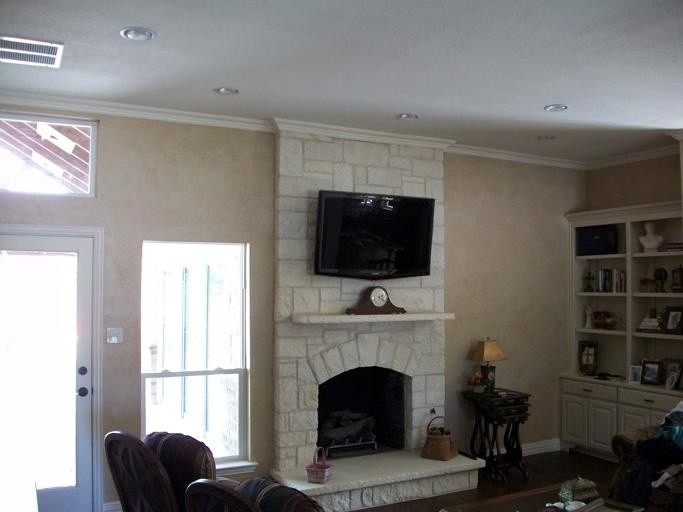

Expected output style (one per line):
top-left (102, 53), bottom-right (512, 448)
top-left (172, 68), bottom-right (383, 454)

top-left (104, 429), bottom-right (324, 512)
top-left (609, 401), bottom-right (682, 508)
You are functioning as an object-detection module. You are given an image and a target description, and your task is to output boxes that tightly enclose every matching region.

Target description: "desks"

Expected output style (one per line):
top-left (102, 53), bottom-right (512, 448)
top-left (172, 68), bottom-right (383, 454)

top-left (461, 388), bottom-right (532, 483)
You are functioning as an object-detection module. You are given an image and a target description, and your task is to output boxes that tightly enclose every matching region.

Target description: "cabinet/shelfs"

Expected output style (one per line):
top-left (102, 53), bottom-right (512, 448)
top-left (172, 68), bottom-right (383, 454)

top-left (558, 200), bottom-right (683, 464)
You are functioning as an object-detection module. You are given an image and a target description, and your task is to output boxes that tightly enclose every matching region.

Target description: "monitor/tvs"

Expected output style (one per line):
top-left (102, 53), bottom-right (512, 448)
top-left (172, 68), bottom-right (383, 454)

top-left (314, 189), bottom-right (434, 279)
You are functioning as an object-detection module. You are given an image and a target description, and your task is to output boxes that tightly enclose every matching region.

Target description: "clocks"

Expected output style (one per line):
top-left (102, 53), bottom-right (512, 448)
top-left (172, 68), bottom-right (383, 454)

top-left (346, 285), bottom-right (406, 315)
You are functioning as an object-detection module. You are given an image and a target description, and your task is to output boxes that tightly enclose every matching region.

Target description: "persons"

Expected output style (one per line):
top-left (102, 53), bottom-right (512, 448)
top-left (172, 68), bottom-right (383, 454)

top-left (638, 220), bottom-right (664, 253)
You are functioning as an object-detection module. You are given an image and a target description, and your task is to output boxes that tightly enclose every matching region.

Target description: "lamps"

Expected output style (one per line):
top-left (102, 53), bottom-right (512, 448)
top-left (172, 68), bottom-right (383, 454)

top-left (473, 337), bottom-right (509, 390)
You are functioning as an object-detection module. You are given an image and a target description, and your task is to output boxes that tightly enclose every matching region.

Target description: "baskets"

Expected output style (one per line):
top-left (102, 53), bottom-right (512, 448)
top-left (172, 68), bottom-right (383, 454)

top-left (422, 416), bottom-right (457, 460)
top-left (304, 447), bottom-right (332, 483)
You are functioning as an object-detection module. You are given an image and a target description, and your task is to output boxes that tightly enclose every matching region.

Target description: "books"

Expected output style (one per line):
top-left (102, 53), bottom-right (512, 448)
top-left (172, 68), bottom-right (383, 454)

top-left (484, 391), bottom-right (500, 397)
top-left (489, 387), bottom-right (516, 395)
top-left (636, 310), bottom-right (664, 335)
top-left (596, 267), bottom-right (627, 293)
